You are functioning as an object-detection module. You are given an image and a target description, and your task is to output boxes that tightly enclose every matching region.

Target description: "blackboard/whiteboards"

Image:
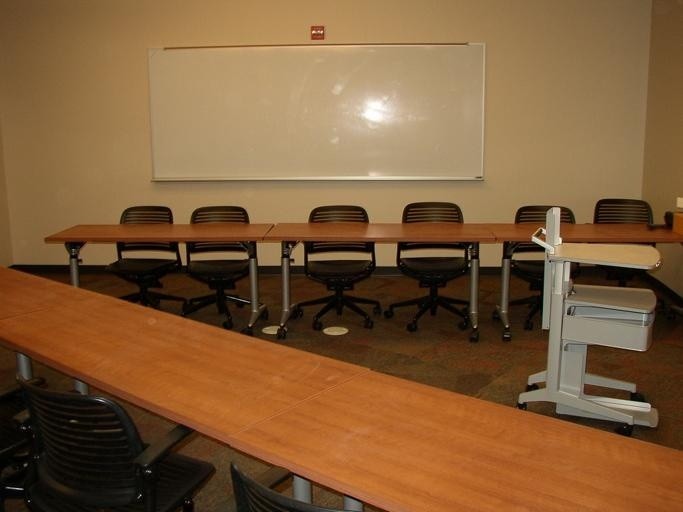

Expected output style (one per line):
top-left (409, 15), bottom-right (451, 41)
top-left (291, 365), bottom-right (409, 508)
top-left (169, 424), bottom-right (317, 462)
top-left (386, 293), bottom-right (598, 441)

top-left (145, 40), bottom-right (487, 182)
